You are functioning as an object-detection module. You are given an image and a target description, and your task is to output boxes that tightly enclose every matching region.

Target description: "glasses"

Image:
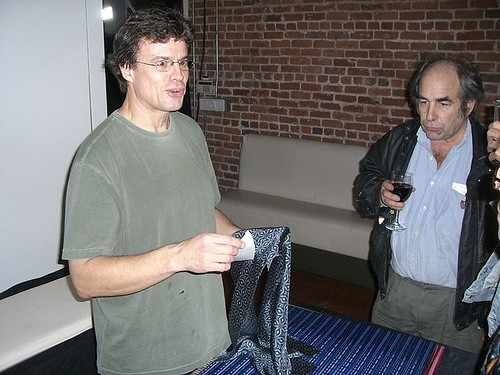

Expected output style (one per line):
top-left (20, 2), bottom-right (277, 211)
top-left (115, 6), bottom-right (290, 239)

top-left (129, 58), bottom-right (194, 73)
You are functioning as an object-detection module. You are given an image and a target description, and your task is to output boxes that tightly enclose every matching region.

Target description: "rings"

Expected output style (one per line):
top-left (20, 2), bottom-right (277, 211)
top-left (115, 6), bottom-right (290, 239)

top-left (384, 198), bottom-right (387, 204)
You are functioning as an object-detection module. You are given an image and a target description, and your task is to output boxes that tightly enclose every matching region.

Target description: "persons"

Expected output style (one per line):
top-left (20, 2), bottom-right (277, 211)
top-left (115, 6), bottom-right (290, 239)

top-left (351, 54), bottom-right (498, 355)
top-left (462, 121), bottom-right (500, 375)
top-left (61, 7), bottom-right (245, 375)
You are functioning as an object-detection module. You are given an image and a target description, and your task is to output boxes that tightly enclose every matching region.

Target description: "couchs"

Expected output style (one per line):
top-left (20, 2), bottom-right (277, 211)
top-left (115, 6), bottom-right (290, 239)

top-left (214, 133), bottom-right (380, 291)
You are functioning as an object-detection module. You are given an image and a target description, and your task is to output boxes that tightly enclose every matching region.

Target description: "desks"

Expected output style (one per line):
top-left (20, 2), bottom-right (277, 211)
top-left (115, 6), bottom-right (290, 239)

top-left (190, 302), bottom-right (500, 374)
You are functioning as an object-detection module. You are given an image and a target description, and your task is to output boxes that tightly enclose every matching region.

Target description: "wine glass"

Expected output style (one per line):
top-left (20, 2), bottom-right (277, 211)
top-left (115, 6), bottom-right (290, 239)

top-left (383, 169), bottom-right (413, 230)
